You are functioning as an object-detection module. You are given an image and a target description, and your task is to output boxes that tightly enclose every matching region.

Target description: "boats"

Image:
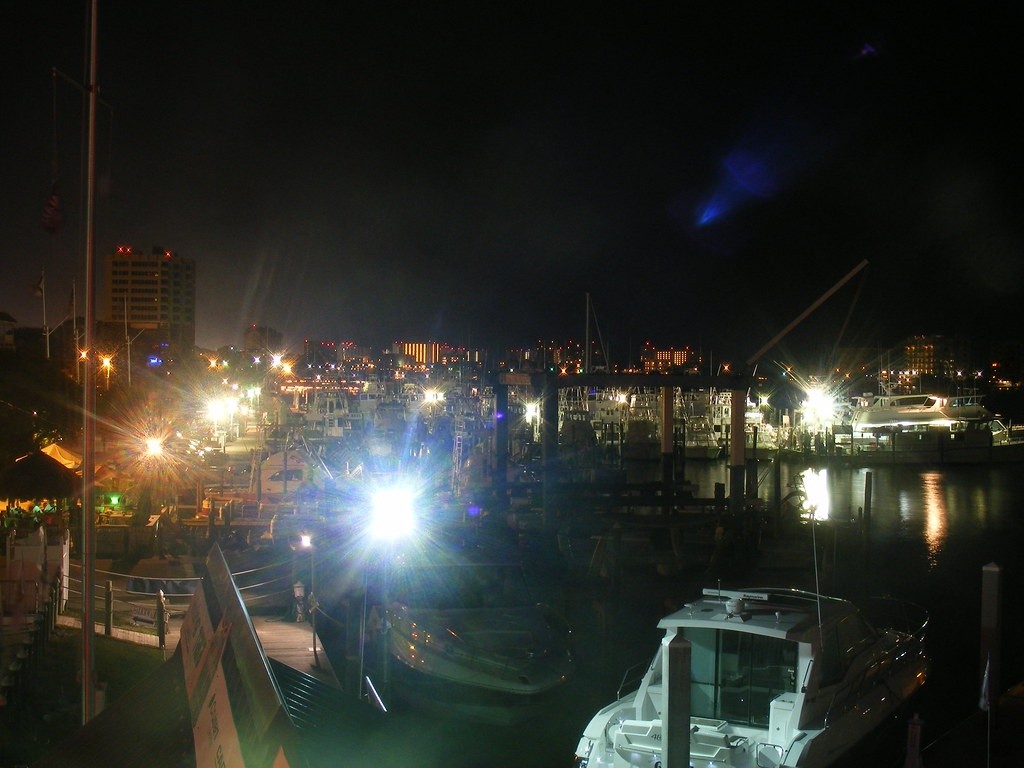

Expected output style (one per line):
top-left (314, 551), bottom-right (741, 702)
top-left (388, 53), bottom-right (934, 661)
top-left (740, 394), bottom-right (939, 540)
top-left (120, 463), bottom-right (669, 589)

top-left (370, 562), bottom-right (573, 695)
top-left (575, 514), bottom-right (930, 768)
top-left (303, 386), bottom-right (792, 461)
top-left (779, 351), bottom-right (1024, 464)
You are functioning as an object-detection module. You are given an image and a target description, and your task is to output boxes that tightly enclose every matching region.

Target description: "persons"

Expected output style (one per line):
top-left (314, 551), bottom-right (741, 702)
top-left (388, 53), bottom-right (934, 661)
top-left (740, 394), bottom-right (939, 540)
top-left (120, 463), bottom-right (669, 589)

top-left (33, 500), bottom-right (56, 523)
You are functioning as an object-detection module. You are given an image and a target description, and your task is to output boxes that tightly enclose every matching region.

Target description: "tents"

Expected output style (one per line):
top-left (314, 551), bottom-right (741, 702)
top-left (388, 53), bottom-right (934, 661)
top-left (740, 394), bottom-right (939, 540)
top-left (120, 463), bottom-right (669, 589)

top-left (0, 444), bottom-right (113, 514)
